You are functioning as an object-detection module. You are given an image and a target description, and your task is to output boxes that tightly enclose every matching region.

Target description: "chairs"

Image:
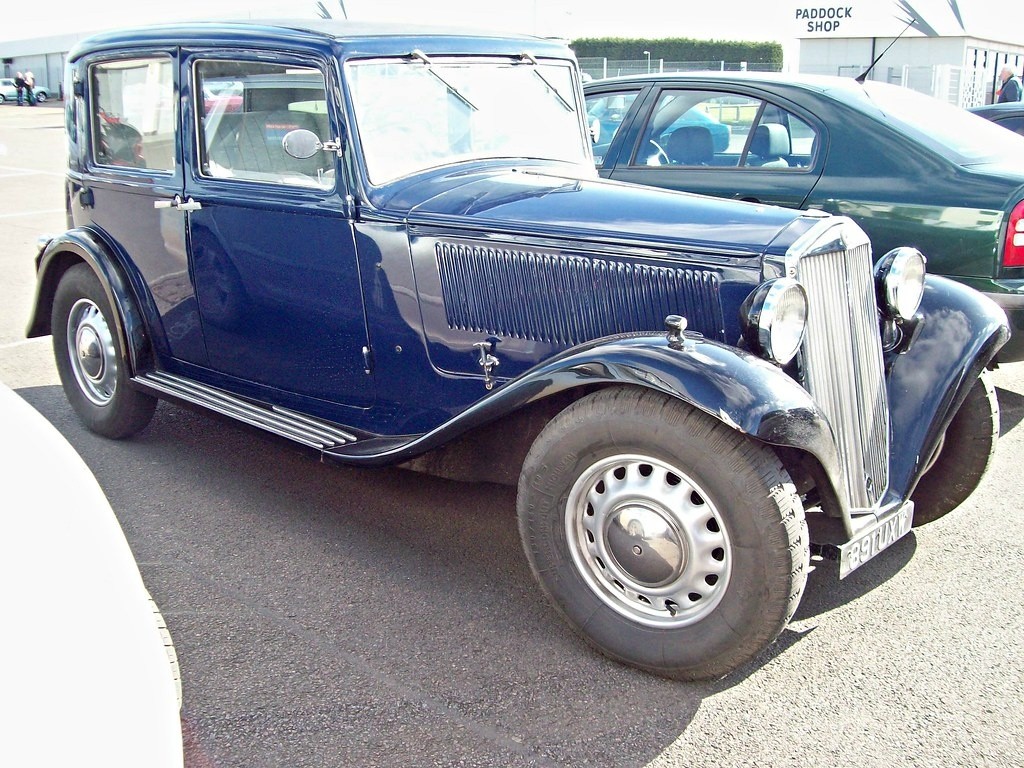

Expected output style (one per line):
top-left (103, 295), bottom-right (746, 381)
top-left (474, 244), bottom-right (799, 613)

top-left (204, 110), bottom-right (328, 179)
top-left (667, 126), bottom-right (715, 167)
top-left (748, 122), bottom-right (791, 169)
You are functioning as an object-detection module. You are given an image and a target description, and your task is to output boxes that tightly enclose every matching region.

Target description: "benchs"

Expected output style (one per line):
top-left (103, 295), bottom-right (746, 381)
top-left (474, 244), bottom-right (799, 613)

top-left (138, 132), bottom-right (175, 170)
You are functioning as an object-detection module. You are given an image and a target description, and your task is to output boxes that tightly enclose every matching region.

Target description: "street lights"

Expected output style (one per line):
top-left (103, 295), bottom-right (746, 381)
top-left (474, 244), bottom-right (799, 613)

top-left (643, 51), bottom-right (650, 74)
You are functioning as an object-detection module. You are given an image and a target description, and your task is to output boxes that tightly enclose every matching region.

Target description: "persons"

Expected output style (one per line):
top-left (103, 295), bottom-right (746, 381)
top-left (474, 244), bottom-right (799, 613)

top-left (24, 70), bottom-right (37, 106)
top-left (14, 72), bottom-right (25, 106)
top-left (997, 63), bottom-right (1023, 103)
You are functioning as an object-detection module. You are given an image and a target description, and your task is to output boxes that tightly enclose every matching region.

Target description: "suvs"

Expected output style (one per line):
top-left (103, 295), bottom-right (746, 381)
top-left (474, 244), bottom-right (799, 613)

top-left (24, 15), bottom-right (1006, 684)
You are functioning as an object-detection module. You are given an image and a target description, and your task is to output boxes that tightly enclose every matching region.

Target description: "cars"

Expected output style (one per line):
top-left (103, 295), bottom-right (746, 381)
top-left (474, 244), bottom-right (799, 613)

top-left (585, 94), bottom-right (733, 163)
top-left (967, 100), bottom-right (1024, 136)
top-left (581, 69), bottom-right (1024, 313)
top-left (200, 86), bottom-right (245, 114)
top-left (0, 77), bottom-right (51, 104)
top-left (202, 110), bottom-right (328, 177)
